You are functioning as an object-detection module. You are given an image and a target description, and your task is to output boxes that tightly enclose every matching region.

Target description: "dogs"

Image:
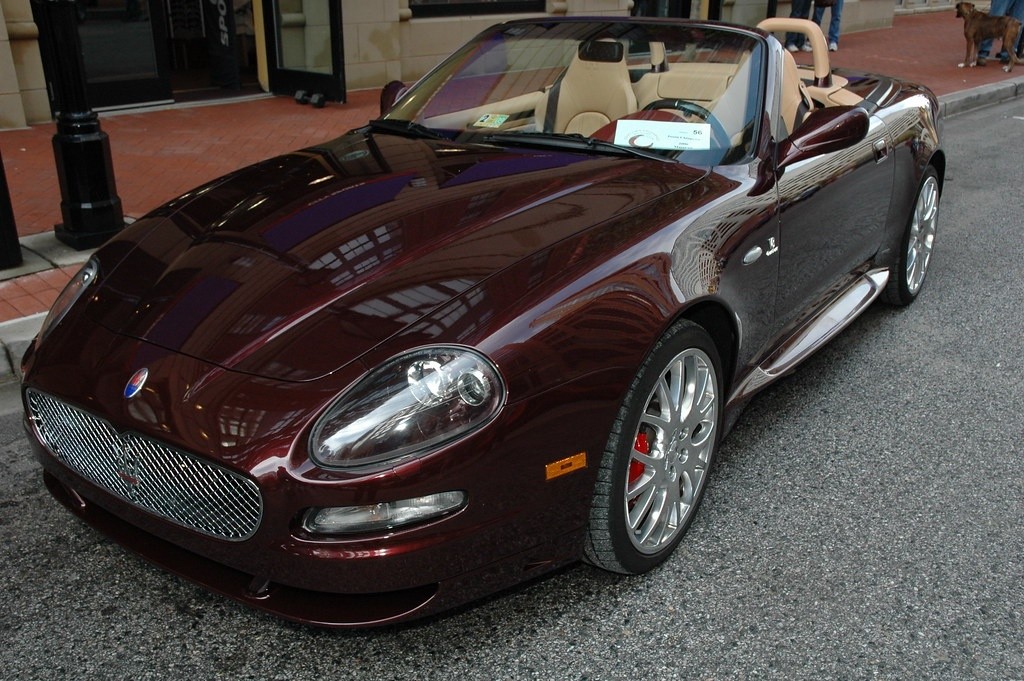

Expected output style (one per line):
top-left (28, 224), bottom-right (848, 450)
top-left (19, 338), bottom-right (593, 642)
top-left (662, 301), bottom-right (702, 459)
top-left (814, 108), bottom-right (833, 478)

top-left (955, 2), bottom-right (1024, 73)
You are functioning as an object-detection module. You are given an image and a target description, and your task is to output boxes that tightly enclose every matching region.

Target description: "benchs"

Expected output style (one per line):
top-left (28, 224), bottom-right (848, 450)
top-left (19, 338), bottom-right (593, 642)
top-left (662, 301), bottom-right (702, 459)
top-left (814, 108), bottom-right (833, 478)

top-left (631, 35), bottom-right (736, 120)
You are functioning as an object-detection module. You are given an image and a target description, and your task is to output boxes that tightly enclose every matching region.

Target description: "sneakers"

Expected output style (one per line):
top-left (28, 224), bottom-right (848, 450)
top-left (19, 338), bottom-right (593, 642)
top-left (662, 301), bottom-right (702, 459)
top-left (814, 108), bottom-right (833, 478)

top-left (801, 45), bottom-right (812, 52)
top-left (829, 42), bottom-right (837, 51)
top-left (787, 44), bottom-right (798, 52)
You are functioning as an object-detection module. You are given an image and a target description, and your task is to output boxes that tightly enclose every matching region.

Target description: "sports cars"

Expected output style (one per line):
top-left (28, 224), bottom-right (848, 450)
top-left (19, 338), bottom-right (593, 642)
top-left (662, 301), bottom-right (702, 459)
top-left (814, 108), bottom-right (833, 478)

top-left (19, 14), bottom-right (947, 632)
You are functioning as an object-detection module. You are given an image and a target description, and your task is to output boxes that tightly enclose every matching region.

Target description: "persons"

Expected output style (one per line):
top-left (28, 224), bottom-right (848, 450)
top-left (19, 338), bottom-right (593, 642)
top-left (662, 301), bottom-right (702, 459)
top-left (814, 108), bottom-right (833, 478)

top-left (480, 115), bottom-right (489, 122)
top-left (978, 0), bottom-right (1024, 66)
top-left (785, 0), bottom-right (843, 52)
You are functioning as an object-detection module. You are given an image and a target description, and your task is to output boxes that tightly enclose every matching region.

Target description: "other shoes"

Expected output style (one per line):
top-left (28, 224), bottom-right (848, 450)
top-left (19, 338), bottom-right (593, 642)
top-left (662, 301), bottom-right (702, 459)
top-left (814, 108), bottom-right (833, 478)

top-left (1000, 57), bottom-right (1009, 64)
top-left (977, 57), bottom-right (986, 66)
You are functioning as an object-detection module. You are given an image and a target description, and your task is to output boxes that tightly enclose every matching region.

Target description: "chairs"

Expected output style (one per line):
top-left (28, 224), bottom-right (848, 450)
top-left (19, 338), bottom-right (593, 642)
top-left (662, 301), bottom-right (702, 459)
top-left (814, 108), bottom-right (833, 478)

top-left (535, 38), bottom-right (638, 138)
top-left (685, 46), bottom-right (815, 150)
